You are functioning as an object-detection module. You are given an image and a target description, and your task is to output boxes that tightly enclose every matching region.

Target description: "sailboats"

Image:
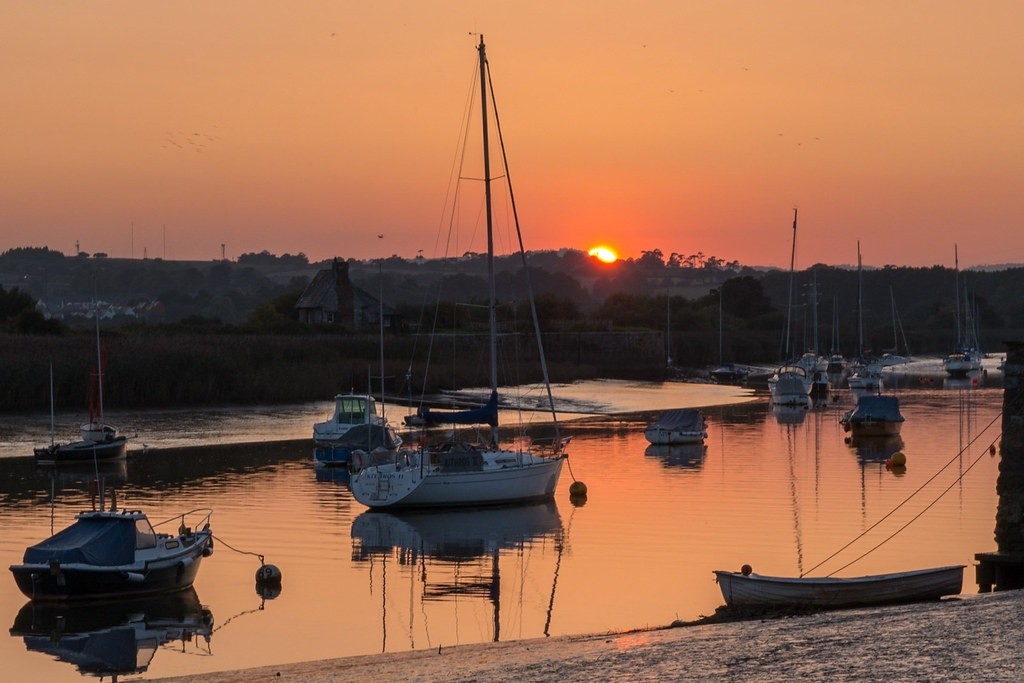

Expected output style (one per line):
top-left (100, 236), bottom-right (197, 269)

top-left (944, 243), bottom-right (982, 377)
top-left (350, 497), bottom-right (566, 652)
top-left (772, 403), bottom-right (812, 578)
top-left (348, 33), bottom-right (573, 511)
top-left (711, 207), bottom-right (908, 403)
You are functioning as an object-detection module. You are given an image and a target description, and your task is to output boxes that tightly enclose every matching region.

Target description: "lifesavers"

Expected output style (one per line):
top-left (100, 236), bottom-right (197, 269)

top-left (346, 449), bottom-right (366, 476)
top-left (399, 449), bottom-right (417, 468)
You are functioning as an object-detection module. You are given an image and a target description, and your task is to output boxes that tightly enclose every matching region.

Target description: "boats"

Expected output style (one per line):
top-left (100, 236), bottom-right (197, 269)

top-left (644, 409), bottom-right (708, 444)
top-left (8, 507), bottom-right (213, 602)
top-left (844, 434), bottom-right (904, 465)
top-left (8, 585), bottom-right (215, 678)
top-left (840, 394), bottom-right (905, 435)
top-left (35, 459), bottom-right (129, 537)
top-left (712, 564), bottom-right (970, 615)
top-left (33, 316), bottom-right (127, 464)
top-left (313, 387), bottom-right (403, 465)
